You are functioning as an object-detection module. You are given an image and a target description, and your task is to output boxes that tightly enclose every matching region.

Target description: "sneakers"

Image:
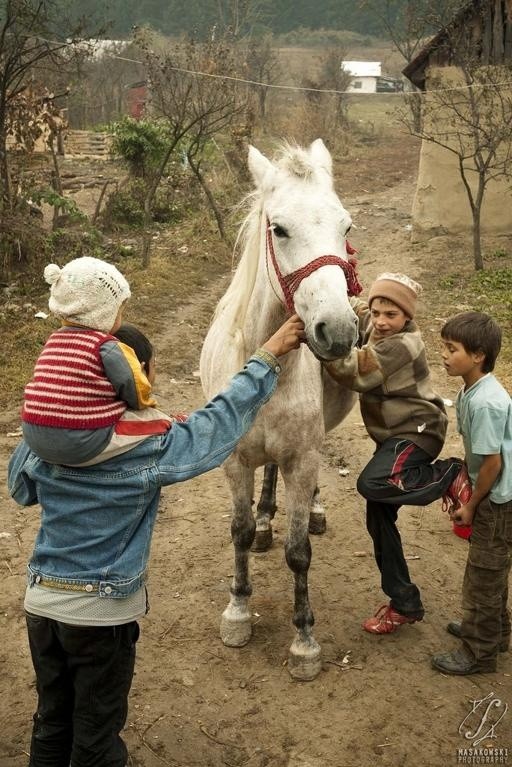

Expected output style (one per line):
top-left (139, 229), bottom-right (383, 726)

top-left (361, 604), bottom-right (424, 634)
top-left (442, 463), bottom-right (472, 521)
top-left (431, 645), bottom-right (496, 674)
top-left (447, 619), bottom-right (509, 652)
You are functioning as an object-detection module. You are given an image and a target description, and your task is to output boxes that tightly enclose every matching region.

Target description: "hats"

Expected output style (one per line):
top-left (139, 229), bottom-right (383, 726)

top-left (44, 256), bottom-right (131, 335)
top-left (368, 273), bottom-right (423, 319)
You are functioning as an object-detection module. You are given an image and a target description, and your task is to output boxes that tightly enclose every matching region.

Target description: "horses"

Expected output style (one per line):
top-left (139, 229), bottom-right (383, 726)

top-left (198, 138), bottom-right (362, 682)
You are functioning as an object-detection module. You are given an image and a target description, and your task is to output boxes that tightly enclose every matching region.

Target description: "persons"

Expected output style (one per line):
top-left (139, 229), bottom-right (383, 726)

top-left (7, 310), bottom-right (309, 767)
top-left (320, 270), bottom-right (473, 633)
top-left (22, 253), bottom-right (189, 470)
top-left (430, 310), bottom-right (511, 676)
